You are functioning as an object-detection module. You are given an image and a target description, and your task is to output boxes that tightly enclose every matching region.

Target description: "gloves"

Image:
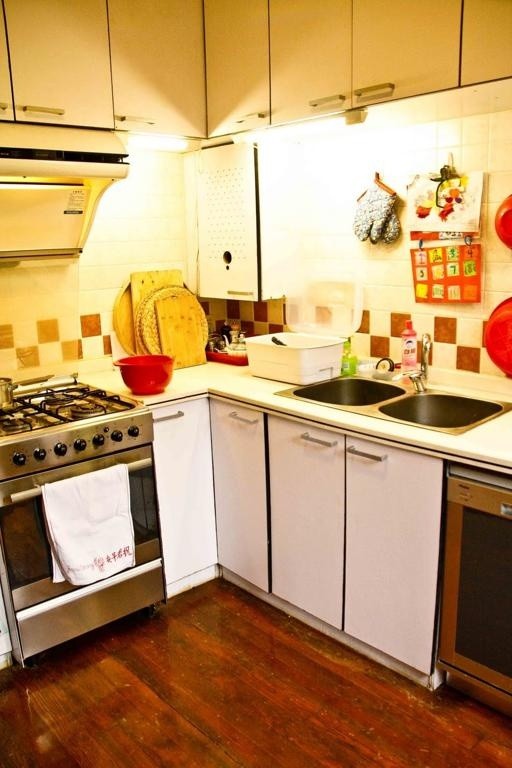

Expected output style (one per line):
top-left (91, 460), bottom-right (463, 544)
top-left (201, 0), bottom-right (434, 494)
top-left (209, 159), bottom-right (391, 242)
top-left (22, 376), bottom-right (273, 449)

top-left (352, 179), bottom-right (401, 244)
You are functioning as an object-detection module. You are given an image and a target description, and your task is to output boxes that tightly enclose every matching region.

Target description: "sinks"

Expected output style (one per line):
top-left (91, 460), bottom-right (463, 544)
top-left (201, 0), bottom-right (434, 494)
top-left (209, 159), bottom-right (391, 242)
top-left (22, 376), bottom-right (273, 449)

top-left (275, 376), bottom-right (407, 416)
top-left (378, 390), bottom-right (512, 435)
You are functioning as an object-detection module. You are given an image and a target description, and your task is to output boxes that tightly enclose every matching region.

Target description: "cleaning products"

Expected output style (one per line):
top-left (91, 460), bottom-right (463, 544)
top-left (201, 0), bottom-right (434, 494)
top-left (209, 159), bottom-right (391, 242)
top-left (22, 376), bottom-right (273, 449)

top-left (401, 319), bottom-right (417, 386)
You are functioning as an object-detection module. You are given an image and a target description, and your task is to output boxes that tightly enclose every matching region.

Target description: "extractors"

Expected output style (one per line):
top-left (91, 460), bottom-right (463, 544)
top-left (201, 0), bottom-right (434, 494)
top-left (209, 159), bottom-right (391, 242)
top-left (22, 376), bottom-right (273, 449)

top-left (2, 124), bottom-right (132, 262)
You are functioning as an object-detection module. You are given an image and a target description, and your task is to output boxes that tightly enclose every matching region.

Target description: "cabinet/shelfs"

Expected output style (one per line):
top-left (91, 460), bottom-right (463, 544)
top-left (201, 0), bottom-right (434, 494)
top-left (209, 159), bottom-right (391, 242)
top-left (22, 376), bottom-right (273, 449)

top-left (457, 0), bottom-right (512, 115)
top-left (263, 0), bottom-right (462, 129)
top-left (103, 1), bottom-right (206, 142)
top-left (149, 395), bottom-right (219, 600)
top-left (260, 409), bottom-right (443, 694)
top-left (207, 395), bottom-right (270, 611)
top-left (187, 140), bottom-right (298, 306)
top-left (0, 1), bottom-right (117, 135)
top-left (197, 0), bottom-right (271, 140)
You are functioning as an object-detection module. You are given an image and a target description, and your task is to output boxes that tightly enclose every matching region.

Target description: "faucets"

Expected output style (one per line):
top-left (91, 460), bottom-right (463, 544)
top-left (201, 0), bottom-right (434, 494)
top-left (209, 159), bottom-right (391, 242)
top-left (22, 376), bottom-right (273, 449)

top-left (421, 332), bottom-right (431, 379)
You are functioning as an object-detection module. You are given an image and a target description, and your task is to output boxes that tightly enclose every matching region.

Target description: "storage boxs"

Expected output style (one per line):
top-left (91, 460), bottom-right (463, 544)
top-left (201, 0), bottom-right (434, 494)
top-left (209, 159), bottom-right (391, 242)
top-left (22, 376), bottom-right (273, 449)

top-left (243, 328), bottom-right (346, 387)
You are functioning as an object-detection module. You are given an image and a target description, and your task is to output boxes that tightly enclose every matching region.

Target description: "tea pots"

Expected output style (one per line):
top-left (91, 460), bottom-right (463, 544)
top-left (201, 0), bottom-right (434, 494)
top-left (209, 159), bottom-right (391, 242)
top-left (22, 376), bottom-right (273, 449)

top-left (222, 332), bottom-right (248, 357)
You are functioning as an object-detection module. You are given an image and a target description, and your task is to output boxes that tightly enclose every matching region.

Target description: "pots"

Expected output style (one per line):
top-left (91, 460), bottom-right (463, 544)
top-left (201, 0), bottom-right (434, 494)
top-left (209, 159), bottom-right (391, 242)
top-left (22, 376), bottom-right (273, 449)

top-left (1, 378), bottom-right (18, 407)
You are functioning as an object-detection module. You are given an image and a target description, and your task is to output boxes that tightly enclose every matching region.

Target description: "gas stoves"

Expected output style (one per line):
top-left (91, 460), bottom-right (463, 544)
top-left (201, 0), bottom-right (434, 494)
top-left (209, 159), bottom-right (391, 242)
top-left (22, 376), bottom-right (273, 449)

top-left (1, 383), bottom-right (157, 482)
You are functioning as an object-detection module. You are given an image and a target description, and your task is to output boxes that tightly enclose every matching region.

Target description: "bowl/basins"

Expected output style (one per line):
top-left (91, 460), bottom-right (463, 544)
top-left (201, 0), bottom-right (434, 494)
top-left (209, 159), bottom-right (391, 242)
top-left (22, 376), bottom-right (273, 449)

top-left (492, 192), bottom-right (511, 250)
top-left (484, 296), bottom-right (512, 380)
top-left (111, 352), bottom-right (176, 396)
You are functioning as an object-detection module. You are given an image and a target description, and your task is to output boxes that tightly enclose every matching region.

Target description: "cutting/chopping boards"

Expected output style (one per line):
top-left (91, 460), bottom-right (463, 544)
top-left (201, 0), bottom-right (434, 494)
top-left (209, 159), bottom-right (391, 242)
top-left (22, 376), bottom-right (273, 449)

top-left (129, 268), bottom-right (207, 370)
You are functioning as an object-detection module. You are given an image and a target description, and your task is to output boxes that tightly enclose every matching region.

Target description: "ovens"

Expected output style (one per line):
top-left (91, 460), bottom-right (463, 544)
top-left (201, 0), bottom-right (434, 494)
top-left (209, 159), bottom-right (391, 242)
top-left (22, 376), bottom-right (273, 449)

top-left (0, 442), bottom-right (164, 670)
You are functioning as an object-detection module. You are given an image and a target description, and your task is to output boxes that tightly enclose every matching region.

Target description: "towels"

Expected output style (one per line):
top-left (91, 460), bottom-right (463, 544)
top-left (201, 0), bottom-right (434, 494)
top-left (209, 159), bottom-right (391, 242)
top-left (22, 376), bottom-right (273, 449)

top-left (40, 463), bottom-right (136, 586)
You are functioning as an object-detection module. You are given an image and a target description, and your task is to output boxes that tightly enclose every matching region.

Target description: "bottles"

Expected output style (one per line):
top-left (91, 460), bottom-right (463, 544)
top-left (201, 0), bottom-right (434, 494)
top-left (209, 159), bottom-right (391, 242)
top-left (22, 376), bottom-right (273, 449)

top-left (401, 320), bottom-right (417, 373)
top-left (208, 316), bottom-right (241, 358)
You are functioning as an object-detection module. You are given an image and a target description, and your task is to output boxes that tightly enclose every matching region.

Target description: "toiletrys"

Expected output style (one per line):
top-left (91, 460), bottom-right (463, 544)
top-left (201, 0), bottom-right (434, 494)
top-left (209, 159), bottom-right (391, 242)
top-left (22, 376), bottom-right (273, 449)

top-left (341, 341), bottom-right (357, 375)
